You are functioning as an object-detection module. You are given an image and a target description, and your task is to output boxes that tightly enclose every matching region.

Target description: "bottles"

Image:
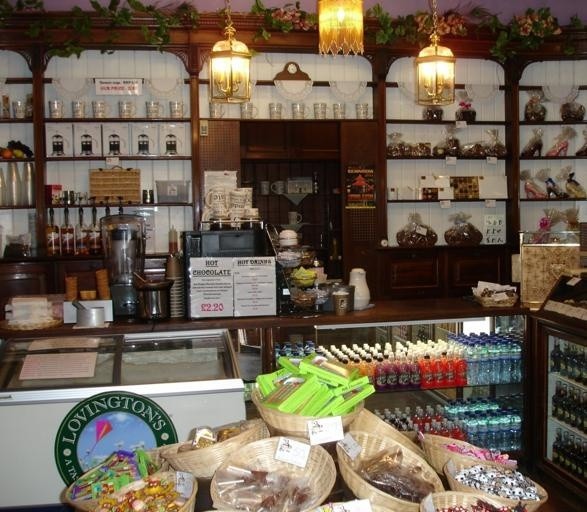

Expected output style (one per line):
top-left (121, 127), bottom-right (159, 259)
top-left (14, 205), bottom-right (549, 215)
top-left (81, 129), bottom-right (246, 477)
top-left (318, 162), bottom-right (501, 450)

top-left (46, 222), bottom-right (103, 257)
top-left (312, 171), bottom-right (320, 194)
top-left (348, 268), bottom-right (371, 311)
top-left (276, 330), bottom-right (521, 391)
top-left (373, 393), bottom-right (522, 454)
top-left (547, 335), bottom-right (586, 480)
top-left (167, 225), bottom-right (177, 253)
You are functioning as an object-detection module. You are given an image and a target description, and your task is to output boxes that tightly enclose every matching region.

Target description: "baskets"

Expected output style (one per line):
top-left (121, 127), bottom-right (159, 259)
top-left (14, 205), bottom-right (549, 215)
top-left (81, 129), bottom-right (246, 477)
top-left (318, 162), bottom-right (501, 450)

top-left (336, 429), bottom-right (447, 512)
top-left (251, 374), bottom-right (365, 440)
top-left (418, 431), bottom-right (519, 477)
top-left (97, 471), bottom-right (199, 512)
top-left (0, 317), bottom-right (63, 330)
top-left (442, 457), bottom-right (550, 512)
top-left (64, 449), bottom-right (171, 512)
top-left (474, 292), bottom-right (518, 307)
top-left (419, 490), bottom-right (513, 512)
top-left (162, 418), bottom-right (270, 480)
top-left (348, 408), bottom-right (427, 460)
top-left (209, 435), bottom-right (336, 512)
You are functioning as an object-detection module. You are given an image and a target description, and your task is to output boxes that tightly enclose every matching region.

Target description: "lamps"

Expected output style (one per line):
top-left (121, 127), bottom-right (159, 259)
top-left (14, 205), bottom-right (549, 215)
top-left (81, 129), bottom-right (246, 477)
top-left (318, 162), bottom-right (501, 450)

top-left (411, 0), bottom-right (458, 108)
top-left (206, 0), bottom-right (256, 104)
top-left (318, 0), bottom-right (366, 57)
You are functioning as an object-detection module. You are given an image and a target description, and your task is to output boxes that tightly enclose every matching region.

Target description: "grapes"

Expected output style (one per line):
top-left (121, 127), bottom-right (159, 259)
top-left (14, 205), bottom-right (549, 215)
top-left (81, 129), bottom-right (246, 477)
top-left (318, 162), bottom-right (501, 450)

top-left (8, 140), bottom-right (33, 158)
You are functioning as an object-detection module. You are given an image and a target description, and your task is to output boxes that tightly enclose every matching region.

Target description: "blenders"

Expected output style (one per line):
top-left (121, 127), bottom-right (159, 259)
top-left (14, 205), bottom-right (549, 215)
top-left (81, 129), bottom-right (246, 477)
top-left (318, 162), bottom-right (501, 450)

top-left (99, 213), bottom-right (148, 322)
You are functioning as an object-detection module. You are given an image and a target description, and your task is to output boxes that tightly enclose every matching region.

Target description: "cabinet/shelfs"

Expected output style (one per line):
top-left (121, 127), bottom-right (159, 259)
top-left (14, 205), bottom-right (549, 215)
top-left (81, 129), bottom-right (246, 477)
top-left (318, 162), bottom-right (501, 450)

top-left (0, 113), bottom-right (586, 263)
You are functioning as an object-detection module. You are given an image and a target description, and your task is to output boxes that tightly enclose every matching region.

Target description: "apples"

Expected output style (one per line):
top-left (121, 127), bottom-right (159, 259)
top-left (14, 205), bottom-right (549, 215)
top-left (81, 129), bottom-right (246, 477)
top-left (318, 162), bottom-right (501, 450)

top-left (2, 149), bottom-right (13, 159)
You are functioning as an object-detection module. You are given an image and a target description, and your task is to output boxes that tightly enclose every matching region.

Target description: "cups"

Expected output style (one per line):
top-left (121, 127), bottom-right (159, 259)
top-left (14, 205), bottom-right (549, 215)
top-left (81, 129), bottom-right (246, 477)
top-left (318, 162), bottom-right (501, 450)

top-left (11, 99), bottom-right (28, 119)
top-left (287, 211), bottom-right (302, 225)
top-left (142, 279), bottom-right (170, 320)
top-left (49, 99), bottom-right (186, 122)
top-left (165, 255), bottom-right (186, 318)
top-left (0, 160), bottom-right (35, 207)
top-left (334, 291), bottom-right (351, 316)
top-left (257, 179), bottom-right (286, 195)
top-left (387, 186), bottom-right (398, 201)
top-left (209, 103), bottom-right (371, 120)
top-left (76, 306), bottom-right (104, 328)
